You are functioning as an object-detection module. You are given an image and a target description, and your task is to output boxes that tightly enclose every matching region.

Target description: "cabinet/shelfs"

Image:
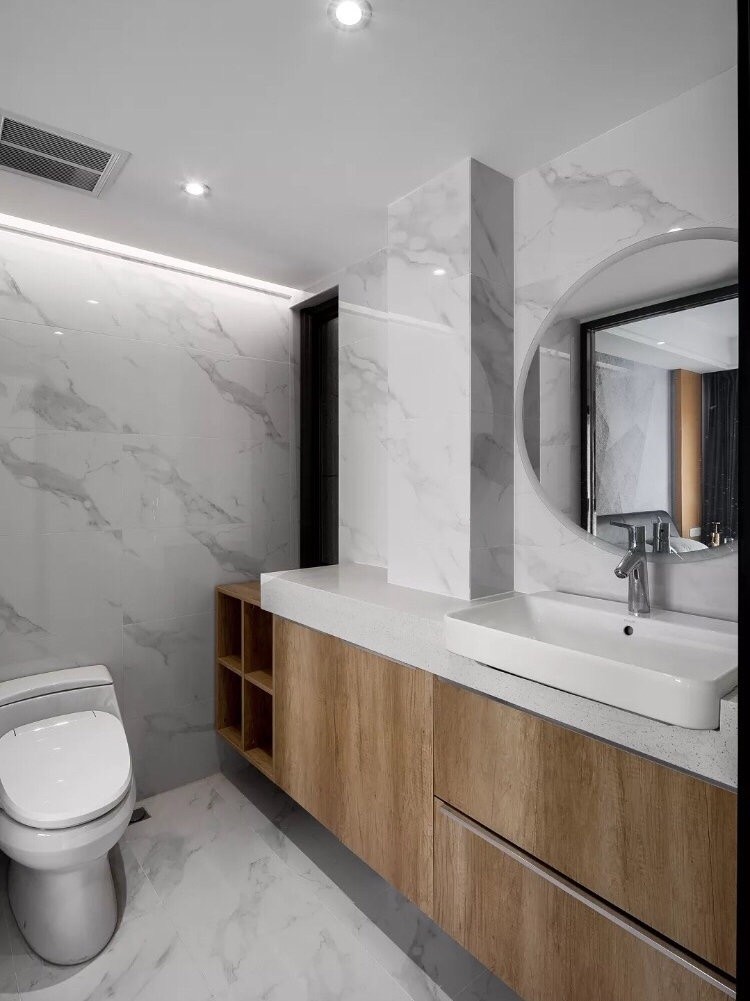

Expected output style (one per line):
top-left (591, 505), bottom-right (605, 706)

top-left (213, 579), bottom-right (735, 1001)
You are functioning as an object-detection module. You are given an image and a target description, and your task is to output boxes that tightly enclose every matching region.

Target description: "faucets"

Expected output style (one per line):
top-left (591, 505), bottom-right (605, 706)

top-left (610, 520), bottom-right (651, 614)
top-left (645, 515), bottom-right (674, 555)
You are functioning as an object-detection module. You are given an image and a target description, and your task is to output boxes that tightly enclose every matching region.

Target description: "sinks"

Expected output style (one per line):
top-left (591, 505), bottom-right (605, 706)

top-left (444, 588), bottom-right (739, 732)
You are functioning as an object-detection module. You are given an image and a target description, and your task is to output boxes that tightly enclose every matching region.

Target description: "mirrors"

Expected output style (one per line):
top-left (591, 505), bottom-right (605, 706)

top-left (514, 225), bottom-right (738, 563)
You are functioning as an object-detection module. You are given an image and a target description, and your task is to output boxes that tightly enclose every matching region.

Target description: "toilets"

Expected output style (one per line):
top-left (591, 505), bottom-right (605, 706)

top-left (1, 664), bottom-right (137, 967)
